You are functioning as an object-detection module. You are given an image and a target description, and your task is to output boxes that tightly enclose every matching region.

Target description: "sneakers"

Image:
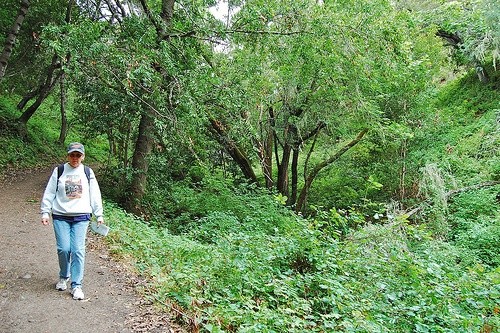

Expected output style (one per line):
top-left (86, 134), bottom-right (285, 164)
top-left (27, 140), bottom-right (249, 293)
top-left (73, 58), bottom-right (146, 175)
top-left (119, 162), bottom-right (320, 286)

top-left (70, 287), bottom-right (84, 300)
top-left (56, 277), bottom-right (69, 291)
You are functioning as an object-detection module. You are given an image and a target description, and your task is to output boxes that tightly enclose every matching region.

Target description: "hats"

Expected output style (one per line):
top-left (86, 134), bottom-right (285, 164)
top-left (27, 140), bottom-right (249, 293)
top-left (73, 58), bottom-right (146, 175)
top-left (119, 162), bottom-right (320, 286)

top-left (66, 142), bottom-right (84, 155)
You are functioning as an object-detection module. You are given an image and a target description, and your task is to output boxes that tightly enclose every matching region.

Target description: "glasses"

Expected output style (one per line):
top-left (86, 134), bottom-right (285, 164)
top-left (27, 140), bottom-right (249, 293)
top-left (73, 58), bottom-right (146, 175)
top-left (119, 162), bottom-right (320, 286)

top-left (70, 153), bottom-right (82, 158)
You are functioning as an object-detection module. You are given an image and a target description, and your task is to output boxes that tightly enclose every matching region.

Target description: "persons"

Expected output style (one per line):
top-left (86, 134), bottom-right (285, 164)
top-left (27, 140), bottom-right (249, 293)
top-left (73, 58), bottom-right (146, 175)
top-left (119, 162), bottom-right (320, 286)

top-left (40, 142), bottom-right (103, 301)
